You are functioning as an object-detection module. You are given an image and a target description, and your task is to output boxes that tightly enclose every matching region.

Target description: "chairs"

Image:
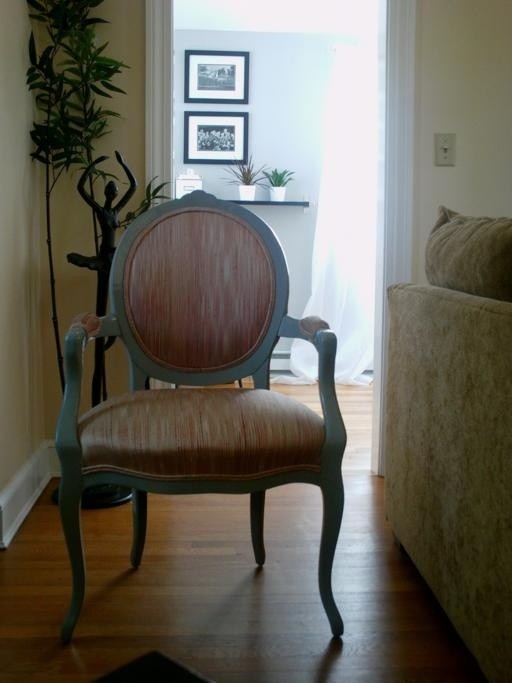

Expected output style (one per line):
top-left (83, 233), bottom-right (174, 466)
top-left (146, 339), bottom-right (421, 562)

top-left (57, 188), bottom-right (346, 649)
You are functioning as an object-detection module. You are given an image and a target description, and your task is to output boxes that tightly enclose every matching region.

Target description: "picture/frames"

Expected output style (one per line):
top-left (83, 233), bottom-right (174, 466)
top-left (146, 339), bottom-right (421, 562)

top-left (183, 49), bottom-right (248, 165)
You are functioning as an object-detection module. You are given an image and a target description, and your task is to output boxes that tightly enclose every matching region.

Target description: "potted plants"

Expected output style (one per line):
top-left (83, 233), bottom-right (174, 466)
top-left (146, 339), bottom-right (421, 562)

top-left (218, 152), bottom-right (297, 202)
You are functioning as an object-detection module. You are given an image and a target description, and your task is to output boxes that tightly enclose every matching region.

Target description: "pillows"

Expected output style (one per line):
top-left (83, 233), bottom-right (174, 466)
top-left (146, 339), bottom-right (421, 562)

top-left (423, 201), bottom-right (512, 301)
